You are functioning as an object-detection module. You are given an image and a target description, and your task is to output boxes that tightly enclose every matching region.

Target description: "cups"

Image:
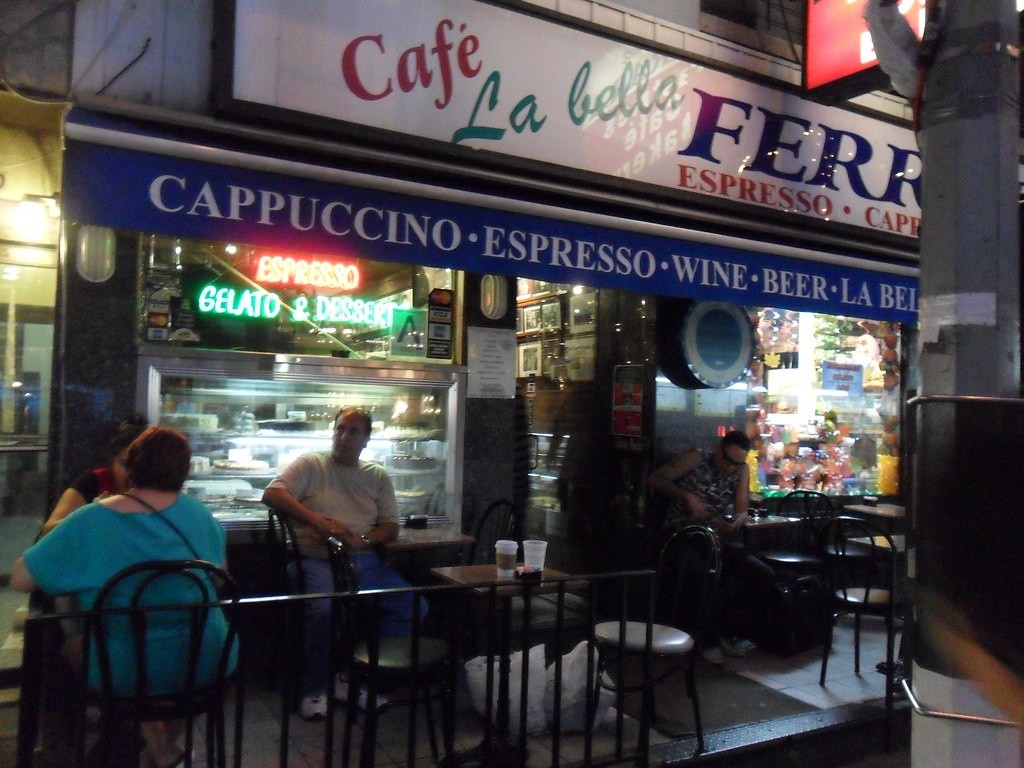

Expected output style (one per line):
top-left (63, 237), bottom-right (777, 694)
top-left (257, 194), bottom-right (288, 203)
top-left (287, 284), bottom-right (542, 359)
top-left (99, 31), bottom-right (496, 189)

top-left (759, 508), bottom-right (768, 518)
top-left (494, 540), bottom-right (519, 582)
top-left (522, 540), bottom-right (548, 581)
top-left (15, 372), bottom-right (41, 435)
top-left (748, 508), bottom-right (758, 517)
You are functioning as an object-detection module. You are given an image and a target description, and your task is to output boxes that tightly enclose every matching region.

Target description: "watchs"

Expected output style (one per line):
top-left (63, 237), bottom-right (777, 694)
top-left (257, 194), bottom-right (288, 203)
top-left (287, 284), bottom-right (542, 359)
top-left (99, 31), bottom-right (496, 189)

top-left (361, 534), bottom-right (370, 545)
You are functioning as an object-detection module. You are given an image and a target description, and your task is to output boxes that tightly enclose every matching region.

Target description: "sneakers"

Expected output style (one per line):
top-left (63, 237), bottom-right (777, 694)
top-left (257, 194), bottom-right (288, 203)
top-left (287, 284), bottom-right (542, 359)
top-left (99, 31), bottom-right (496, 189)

top-left (300, 696), bottom-right (327, 720)
top-left (334, 674), bottom-right (392, 710)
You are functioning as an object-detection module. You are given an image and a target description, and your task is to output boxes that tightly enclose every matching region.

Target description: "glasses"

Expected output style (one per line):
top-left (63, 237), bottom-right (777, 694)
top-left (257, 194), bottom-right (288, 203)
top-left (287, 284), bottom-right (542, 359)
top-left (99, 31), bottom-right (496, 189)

top-left (721, 448), bottom-right (746, 471)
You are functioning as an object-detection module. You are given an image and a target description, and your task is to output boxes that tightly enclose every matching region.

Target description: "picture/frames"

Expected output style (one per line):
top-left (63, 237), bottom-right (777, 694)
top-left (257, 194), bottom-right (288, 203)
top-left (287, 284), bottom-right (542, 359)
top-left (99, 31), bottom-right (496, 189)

top-left (516, 277), bottom-right (597, 385)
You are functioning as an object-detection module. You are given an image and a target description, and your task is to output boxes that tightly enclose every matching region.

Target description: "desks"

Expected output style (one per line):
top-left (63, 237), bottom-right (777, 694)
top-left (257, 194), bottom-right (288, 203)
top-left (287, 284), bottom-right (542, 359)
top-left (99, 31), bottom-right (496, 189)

top-left (847, 535), bottom-right (907, 557)
top-left (430, 562), bottom-right (589, 768)
top-left (378, 525), bottom-right (475, 587)
top-left (729, 511), bottom-right (801, 552)
top-left (843, 502), bottom-right (906, 521)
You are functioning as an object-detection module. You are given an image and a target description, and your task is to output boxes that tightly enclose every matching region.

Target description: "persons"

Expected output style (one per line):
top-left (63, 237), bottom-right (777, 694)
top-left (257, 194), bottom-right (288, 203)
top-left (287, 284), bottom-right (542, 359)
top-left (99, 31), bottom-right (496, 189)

top-left (542, 374), bottom-right (618, 598)
top-left (5, 420), bottom-right (241, 767)
top-left (260, 404), bottom-right (431, 723)
top-left (36, 415), bottom-right (148, 686)
top-left (907, 404), bottom-right (1024, 730)
top-left (647, 430), bottom-right (775, 665)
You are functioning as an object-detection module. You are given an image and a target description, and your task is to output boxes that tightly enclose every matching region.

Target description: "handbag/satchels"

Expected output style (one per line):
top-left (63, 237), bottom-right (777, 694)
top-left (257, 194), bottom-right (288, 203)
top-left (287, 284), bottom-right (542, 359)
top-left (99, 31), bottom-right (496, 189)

top-left (464, 640), bottom-right (617, 737)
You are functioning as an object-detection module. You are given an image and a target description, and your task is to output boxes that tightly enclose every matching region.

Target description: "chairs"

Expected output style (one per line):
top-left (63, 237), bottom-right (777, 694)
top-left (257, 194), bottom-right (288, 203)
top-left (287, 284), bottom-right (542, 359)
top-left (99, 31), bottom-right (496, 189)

top-left (62, 489), bottom-right (907, 768)
